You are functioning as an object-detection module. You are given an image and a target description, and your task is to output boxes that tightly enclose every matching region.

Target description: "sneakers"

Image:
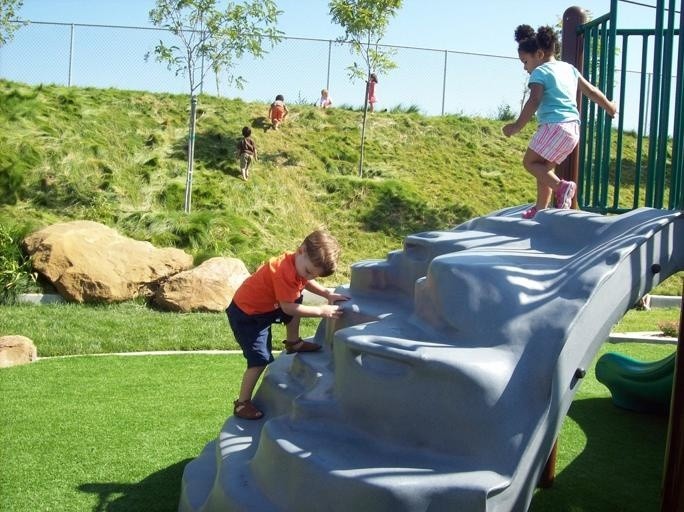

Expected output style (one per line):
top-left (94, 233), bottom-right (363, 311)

top-left (555, 180), bottom-right (576, 209)
top-left (522, 207), bottom-right (537, 219)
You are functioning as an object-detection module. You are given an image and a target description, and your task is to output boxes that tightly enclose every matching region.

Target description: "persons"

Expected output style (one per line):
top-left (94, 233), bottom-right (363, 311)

top-left (320, 88), bottom-right (334, 110)
top-left (502, 23), bottom-right (616, 218)
top-left (236, 126), bottom-right (258, 178)
top-left (365, 74), bottom-right (378, 110)
top-left (268, 95), bottom-right (287, 131)
top-left (226, 230), bottom-right (351, 419)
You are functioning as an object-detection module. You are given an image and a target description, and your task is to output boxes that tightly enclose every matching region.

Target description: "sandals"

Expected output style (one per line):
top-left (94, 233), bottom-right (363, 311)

top-left (282, 338), bottom-right (322, 355)
top-left (234, 400), bottom-right (263, 420)
